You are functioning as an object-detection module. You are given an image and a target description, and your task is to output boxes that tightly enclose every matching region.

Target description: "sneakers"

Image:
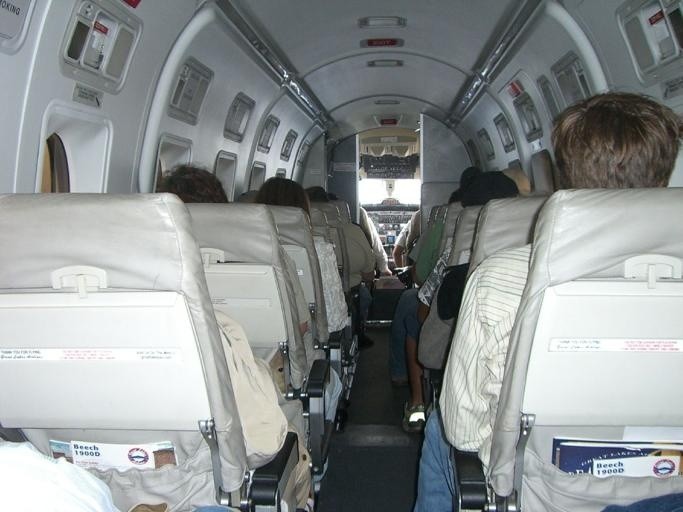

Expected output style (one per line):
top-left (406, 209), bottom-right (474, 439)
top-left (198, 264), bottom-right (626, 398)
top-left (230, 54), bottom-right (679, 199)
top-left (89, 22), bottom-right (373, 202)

top-left (389, 368), bottom-right (410, 388)
top-left (358, 336), bottom-right (374, 349)
top-left (401, 401), bottom-right (426, 435)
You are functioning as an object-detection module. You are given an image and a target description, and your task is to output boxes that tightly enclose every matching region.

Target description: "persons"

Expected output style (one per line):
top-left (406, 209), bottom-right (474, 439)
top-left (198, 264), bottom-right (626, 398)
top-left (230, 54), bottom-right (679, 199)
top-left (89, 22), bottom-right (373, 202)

top-left (413, 87), bottom-right (682, 511)
top-left (153, 163), bottom-right (393, 512)
top-left (392, 163), bottom-right (522, 433)
